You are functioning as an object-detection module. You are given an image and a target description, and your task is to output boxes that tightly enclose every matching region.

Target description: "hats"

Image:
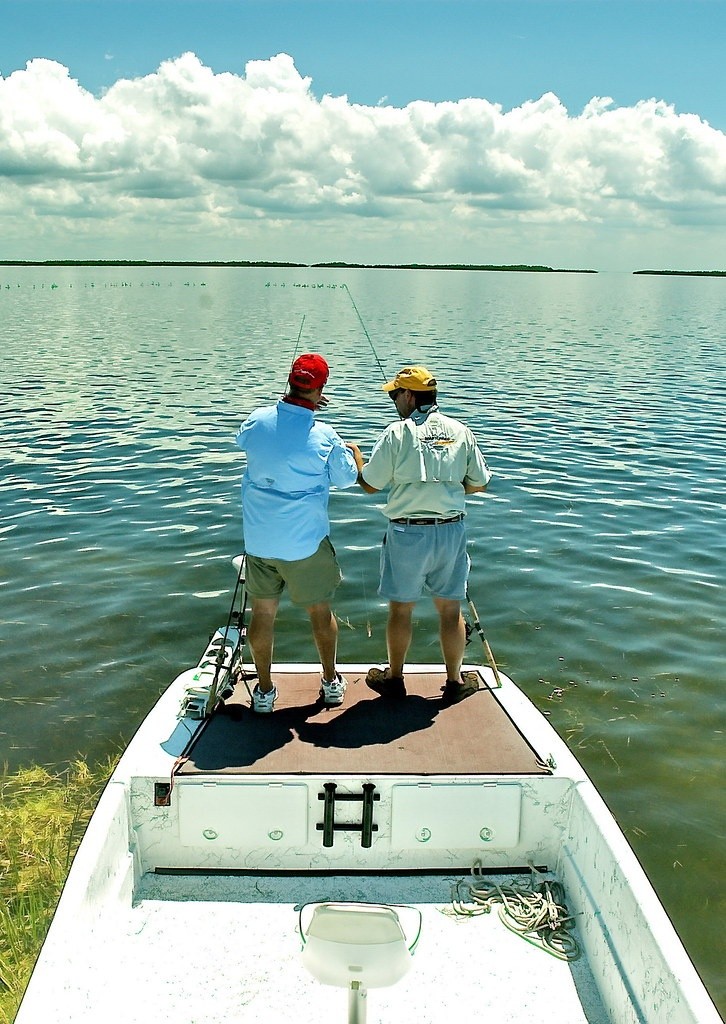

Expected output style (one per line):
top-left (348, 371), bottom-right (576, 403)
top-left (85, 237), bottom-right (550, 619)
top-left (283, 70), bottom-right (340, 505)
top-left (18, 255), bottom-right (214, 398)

top-left (289, 353), bottom-right (329, 390)
top-left (382, 366), bottom-right (437, 391)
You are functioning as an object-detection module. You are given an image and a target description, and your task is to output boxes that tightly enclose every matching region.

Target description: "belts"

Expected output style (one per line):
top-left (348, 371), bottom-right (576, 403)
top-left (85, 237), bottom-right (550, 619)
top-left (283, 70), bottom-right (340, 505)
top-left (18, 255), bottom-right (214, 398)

top-left (390, 513), bottom-right (463, 525)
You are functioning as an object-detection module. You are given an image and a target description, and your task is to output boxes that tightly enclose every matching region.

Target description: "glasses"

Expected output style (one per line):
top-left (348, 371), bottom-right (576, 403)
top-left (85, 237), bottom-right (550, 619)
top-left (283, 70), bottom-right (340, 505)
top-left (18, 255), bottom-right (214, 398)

top-left (388, 389), bottom-right (399, 400)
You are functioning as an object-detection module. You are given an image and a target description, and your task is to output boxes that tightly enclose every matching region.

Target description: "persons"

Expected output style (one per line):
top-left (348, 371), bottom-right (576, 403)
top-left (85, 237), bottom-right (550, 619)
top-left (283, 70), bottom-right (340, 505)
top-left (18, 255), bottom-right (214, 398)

top-left (235, 354), bottom-right (359, 713)
top-left (344, 367), bottom-right (493, 699)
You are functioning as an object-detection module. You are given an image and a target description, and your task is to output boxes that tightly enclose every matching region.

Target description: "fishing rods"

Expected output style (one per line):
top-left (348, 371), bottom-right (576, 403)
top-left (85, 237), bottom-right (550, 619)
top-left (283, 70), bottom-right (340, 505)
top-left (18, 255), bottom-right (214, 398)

top-left (342, 283), bottom-right (501, 687)
top-left (207, 313), bottom-right (305, 717)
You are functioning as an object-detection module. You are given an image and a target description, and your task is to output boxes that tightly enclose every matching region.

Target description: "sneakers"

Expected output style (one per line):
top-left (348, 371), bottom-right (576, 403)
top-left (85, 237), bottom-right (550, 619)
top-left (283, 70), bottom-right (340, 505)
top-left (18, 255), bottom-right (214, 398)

top-left (365, 668), bottom-right (406, 700)
top-left (253, 680), bottom-right (279, 712)
top-left (442, 672), bottom-right (479, 704)
top-left (320, 670), bottom-right (348, 703)
